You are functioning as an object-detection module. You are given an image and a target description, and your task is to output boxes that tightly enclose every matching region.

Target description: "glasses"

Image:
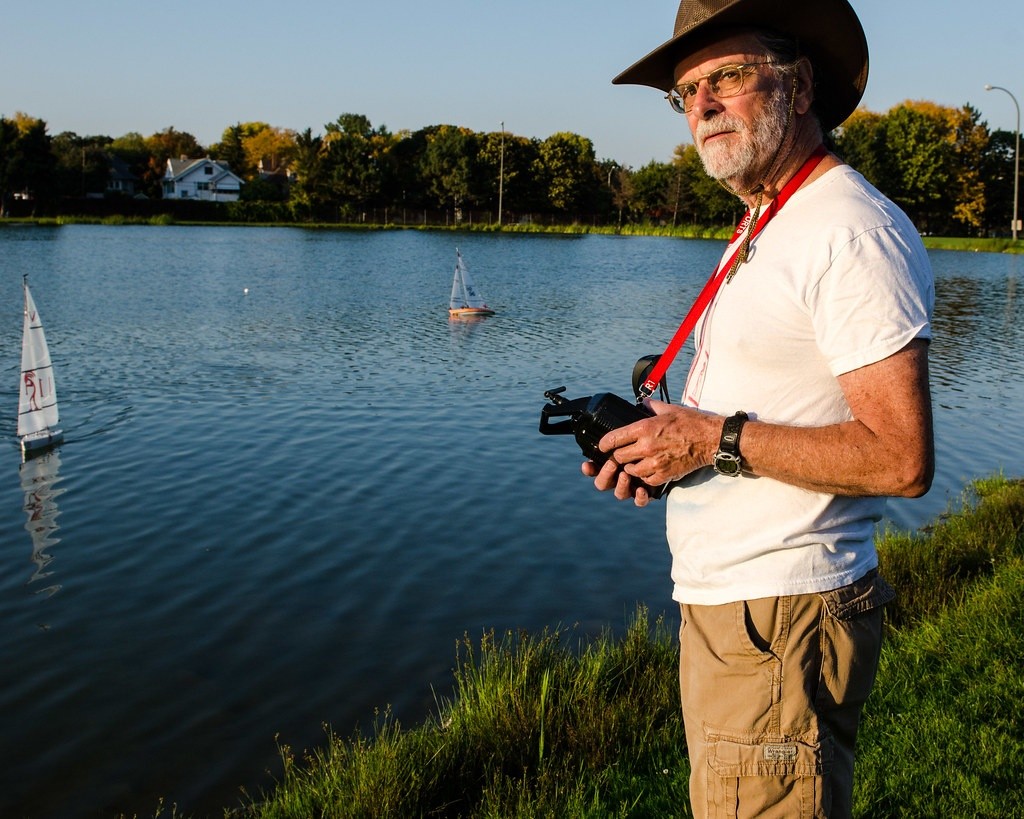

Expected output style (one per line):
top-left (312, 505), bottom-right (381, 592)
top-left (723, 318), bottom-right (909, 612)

top-left (664, 60), bottom-right (793, 114)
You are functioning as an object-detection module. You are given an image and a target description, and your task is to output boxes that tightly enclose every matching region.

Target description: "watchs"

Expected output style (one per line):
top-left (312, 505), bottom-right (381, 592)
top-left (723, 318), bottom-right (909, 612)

top-left (712, 411), bottom-right (749, 478)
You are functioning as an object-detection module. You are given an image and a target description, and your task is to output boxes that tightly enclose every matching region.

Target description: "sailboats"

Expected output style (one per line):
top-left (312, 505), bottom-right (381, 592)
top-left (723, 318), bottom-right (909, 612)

top-left (449, 246), bottom-right (496, 320)
top-left (13, 275), bottom-right (70, 465)
top-left (19, 443), bottom-right (60, 586)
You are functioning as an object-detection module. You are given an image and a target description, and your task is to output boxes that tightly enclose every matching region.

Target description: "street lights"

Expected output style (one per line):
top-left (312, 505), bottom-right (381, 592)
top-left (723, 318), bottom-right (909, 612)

top-left (498, 121), bottom-right (504, 226)
top-left (984, 83), bottom-right (1019, 242)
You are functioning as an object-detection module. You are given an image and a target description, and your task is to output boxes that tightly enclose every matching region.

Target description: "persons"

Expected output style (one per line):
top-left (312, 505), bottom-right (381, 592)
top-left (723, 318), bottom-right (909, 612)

top-left (582, 0), bottom-right (936, 819)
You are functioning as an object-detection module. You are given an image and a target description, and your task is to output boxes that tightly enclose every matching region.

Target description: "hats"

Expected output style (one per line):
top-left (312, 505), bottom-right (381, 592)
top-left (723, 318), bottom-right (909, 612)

top-left (612, 0), bottom-right (869, 133)
